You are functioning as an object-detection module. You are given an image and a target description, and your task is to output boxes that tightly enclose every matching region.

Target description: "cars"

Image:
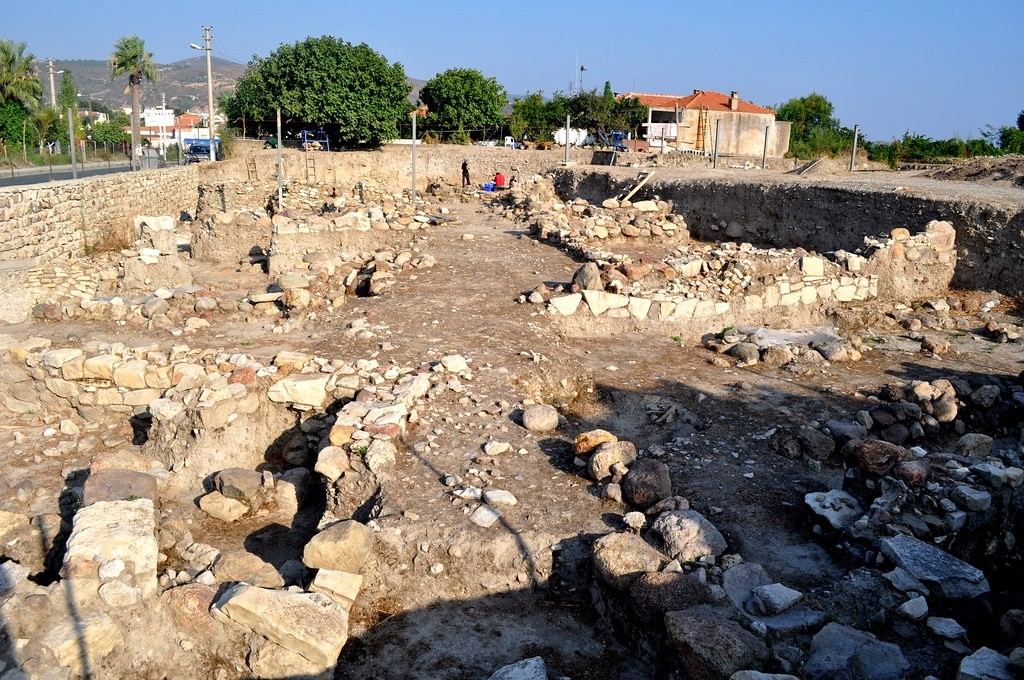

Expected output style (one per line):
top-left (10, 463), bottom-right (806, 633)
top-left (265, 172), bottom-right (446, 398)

top-left (182, 142), bottom-right (210, 164)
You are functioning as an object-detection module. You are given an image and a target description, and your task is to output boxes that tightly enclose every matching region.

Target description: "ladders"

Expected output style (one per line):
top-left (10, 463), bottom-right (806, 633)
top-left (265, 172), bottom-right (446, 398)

top-left (613, 169), bottom-right (657, 201)
top-left (306, 152), bottom-right (316, 182)
top-left (701, 103), bottom-right (714, 156)
top-left (246, 154), bottom-right (259, 179)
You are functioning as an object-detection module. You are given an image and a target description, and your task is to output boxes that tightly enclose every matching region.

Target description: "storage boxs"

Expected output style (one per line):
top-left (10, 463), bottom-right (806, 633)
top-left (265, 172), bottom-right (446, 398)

top-left (485, 183), bottom-right (495, 191)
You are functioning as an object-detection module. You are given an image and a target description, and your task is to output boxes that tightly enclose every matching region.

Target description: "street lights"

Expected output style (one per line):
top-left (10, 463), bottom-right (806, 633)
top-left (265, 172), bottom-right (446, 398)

top-left (190, 43), bottom-right (217, 162)
top-left (50, 69), bottom-right (65, 155)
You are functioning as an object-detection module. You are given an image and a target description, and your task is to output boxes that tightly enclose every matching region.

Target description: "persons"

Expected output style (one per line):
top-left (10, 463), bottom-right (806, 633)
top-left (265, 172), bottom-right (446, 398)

top-left (136, 143), bottom-right (146, 169)
top-left (462, 158), bottom-right (471, 188)
top-left (494, 172), bottom-right (505, 190)
